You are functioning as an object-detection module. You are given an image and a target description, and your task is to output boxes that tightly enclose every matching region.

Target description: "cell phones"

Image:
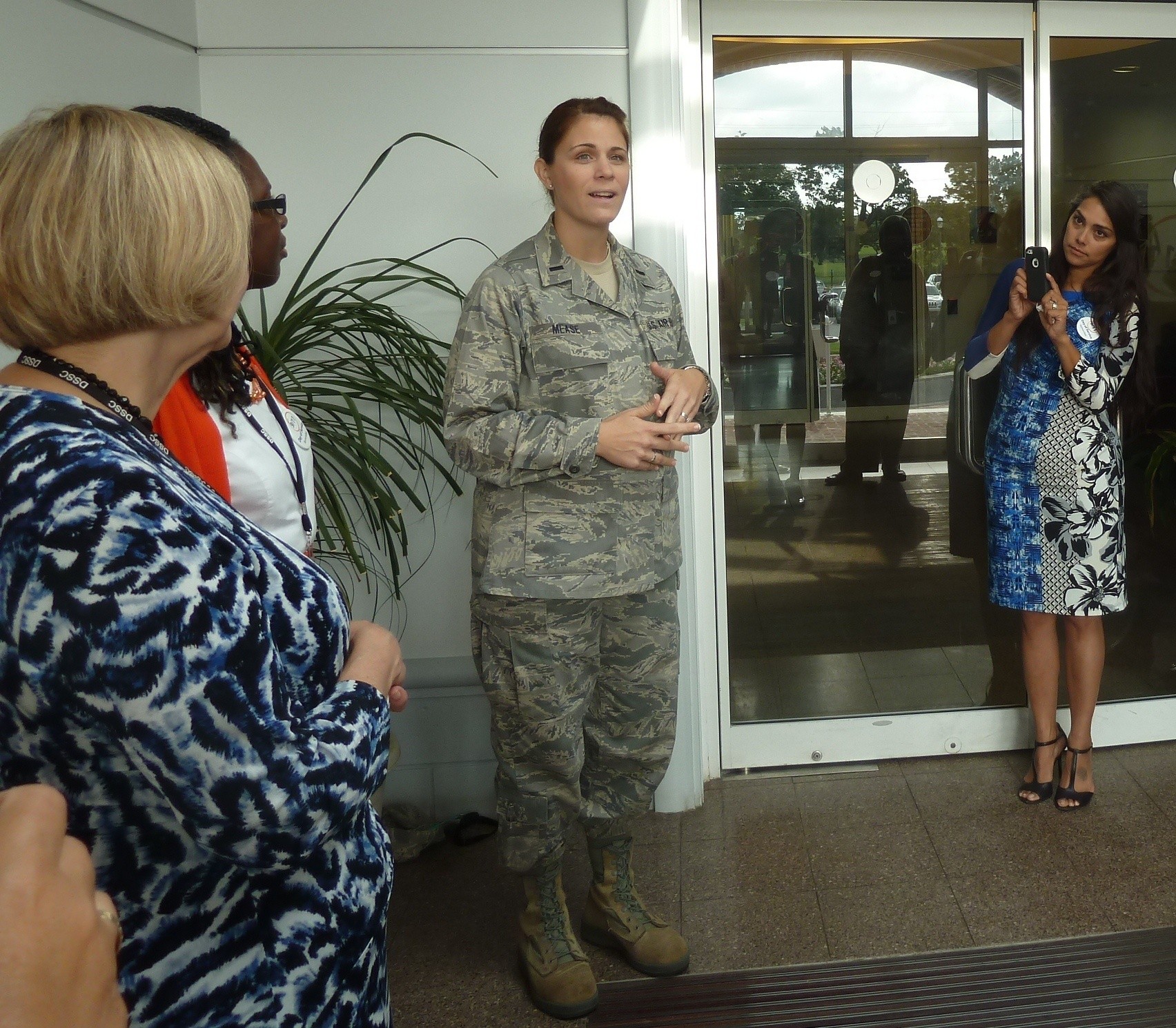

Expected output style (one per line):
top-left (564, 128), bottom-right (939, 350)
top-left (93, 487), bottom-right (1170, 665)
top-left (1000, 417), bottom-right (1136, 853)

top-left (1025, 246), bottom-right (1049, 302)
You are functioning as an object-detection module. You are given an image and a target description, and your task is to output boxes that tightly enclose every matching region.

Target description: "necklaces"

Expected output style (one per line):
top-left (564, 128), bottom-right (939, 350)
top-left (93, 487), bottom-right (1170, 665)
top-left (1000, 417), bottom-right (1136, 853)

top-left (236, 354), bottom-right (266, 404)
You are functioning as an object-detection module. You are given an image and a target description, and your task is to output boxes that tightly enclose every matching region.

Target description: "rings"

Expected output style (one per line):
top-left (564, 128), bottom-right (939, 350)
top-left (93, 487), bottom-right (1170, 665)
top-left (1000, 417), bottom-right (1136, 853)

top-left (650, 451), bottom-right (657, 464)
top-left (1053, 302), bottom-right (1058, 310)
top-left (687, 417), bottom-right (691, 423)
top-left (681, 412), bottom-right (687, 419)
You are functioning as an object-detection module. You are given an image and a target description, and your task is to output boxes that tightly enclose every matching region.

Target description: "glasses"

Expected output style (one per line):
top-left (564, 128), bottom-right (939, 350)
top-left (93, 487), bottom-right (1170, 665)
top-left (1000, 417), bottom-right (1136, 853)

top-left (248, 195), bottom-right (288, 215)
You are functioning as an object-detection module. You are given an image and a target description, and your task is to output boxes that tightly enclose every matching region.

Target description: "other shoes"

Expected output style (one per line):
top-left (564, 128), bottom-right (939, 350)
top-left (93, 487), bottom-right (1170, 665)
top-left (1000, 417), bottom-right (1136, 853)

top-left (767, 481), bottom-right (789, 508)
top-left (825, 471), bottom-right (862, 483)
top-left (882, 466), bottom-right (908, 482)
top-left (787, 480), bottom-right (806, 508)
top-left (764, 464), bottom-right (791, 473)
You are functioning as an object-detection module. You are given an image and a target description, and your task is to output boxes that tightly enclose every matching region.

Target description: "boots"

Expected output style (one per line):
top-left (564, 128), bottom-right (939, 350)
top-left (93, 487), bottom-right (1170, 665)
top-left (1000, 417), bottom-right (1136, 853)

top-left (579, 838), bottom-right (690, 975)
top-left (515, 858), bottom-right (600, 1019)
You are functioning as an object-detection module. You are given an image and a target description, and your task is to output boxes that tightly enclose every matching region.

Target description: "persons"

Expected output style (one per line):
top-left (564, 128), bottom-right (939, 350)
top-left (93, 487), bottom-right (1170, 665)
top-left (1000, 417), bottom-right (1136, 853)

top-left (0, 783), bottom-right (130, 1028)
top-left (440, 93), bottom-right (722, 1018)
top-left (0, 102), bottom-right (413, 1028)
top-left (134, 105), bottom-right (327, 565)
top-left (720, 206), bottom-right (1023, 509)
top-left (964, 176), bottom-right (1147, 809)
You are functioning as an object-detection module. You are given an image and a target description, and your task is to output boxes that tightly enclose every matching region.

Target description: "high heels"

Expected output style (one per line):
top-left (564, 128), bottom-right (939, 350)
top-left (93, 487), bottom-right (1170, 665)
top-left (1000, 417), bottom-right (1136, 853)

top-left (1054, 740), bottom-right (1094, 811)
top-left (1017, 722), bottom-right (1067, 805)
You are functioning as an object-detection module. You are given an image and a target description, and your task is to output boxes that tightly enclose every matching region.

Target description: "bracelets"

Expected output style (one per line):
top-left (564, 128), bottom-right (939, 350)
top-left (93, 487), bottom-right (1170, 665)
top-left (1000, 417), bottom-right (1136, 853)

top-left (684, 365), bottom-right (711, 402)
